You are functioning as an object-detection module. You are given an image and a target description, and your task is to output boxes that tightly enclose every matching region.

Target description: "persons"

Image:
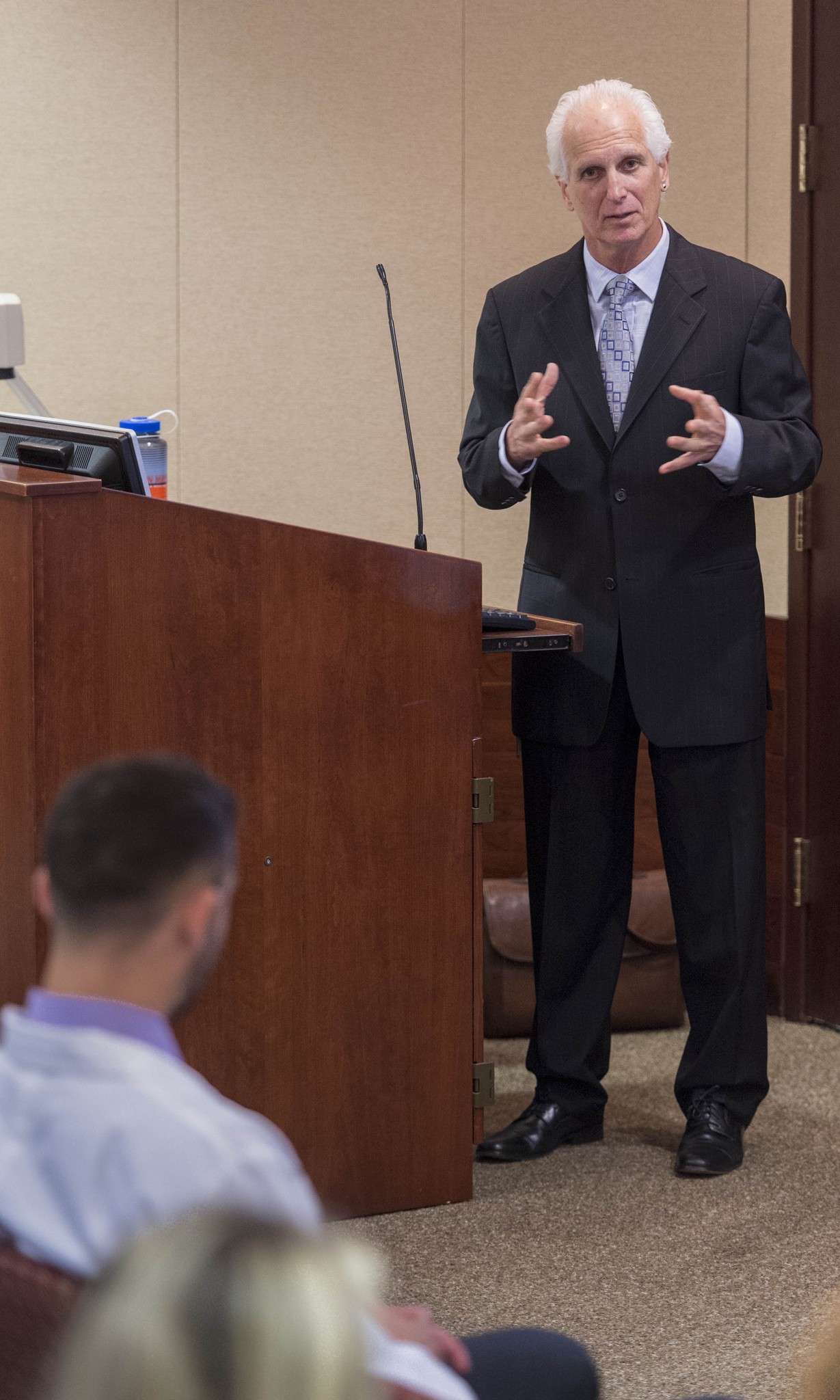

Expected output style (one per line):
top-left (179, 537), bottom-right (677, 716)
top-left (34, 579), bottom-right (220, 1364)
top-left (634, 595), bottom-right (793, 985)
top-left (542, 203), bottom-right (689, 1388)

top-left (457, 79), bottom-right (823, 1176)
top-left (0, 761), bottom-right (602, 1400)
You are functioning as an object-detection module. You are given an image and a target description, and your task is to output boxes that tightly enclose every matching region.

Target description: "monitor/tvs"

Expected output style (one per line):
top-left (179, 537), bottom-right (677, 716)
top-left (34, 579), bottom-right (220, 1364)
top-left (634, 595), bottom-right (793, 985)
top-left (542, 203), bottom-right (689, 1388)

top-left (0, 411), bottom-right (152, 500)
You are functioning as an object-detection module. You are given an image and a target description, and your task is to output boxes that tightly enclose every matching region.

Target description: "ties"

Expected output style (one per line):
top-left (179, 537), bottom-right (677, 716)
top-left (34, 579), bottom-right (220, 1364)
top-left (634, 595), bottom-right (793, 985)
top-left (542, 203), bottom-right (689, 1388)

top-left (597, 274), bottom-right (639, 434)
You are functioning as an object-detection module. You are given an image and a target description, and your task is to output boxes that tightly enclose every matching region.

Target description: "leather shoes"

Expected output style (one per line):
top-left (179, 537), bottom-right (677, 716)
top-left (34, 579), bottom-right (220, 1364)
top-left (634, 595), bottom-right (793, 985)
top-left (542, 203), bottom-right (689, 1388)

top-left (675, 1085), bottom-right (744, 1173)
top-left (477, 1101), bottom-right (604, 1161)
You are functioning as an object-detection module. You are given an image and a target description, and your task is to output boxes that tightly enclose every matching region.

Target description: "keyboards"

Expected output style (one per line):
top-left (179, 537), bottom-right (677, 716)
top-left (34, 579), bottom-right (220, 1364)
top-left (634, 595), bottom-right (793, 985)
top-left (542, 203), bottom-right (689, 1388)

top-left (481, 606), bottom-right (536, 632)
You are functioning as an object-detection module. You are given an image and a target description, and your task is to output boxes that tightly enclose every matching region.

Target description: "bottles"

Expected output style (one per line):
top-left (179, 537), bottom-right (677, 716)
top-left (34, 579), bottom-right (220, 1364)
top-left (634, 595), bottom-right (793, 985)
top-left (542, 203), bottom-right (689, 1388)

top-left (119, 410), bottom-right (179, 500)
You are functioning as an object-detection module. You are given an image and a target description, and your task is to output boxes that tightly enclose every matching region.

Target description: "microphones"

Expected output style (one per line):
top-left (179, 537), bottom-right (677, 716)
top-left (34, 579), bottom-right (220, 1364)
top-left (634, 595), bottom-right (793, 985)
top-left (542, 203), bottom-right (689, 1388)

top-left (376, 263), bottom-right (428, 552)
